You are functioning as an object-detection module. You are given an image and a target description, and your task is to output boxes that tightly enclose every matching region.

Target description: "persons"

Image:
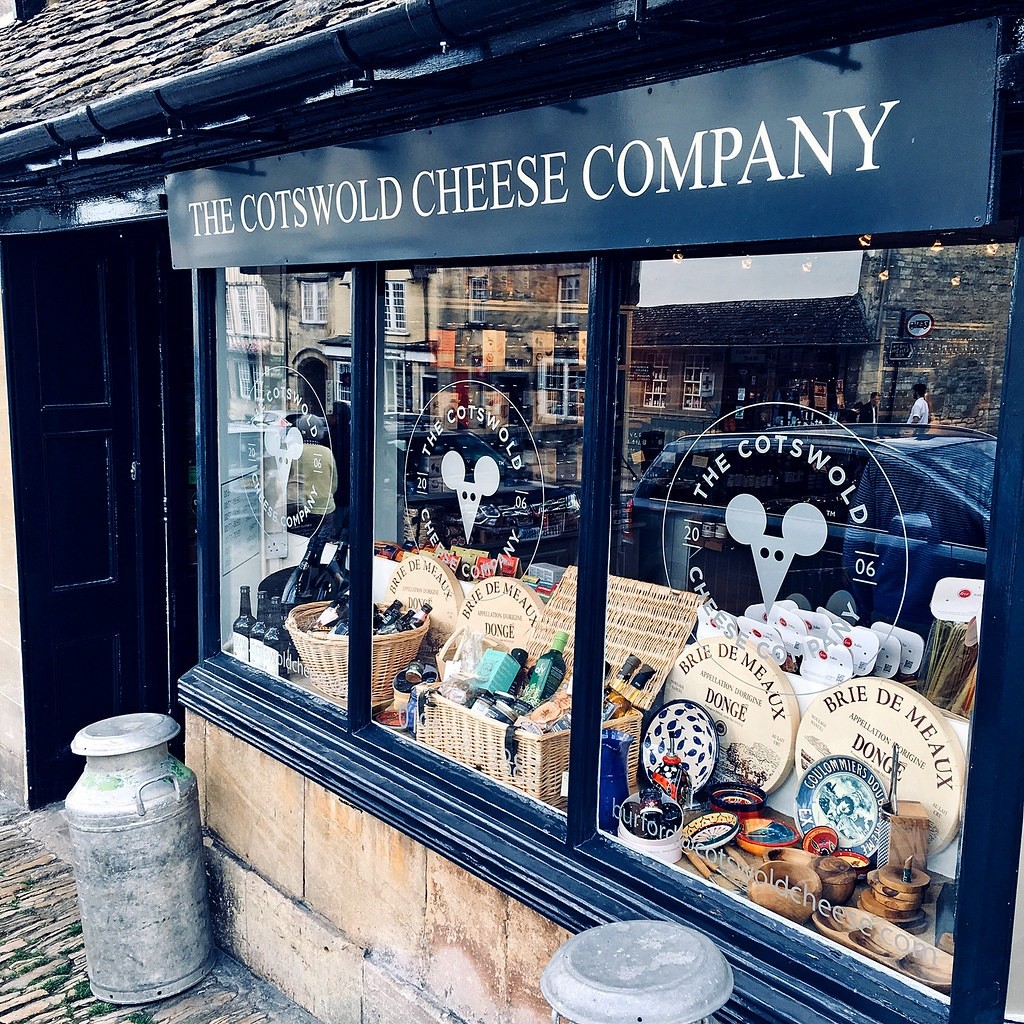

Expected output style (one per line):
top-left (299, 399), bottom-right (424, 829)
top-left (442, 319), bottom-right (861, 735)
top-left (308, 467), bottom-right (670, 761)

top-left (274, 400), bottom-right (350, 544)
top-left (841, 356), bottom-right (996, 634)
top-left (838, 392), bottom-right (881, 437)
top-left (906, 383), bottom-right (930, 434)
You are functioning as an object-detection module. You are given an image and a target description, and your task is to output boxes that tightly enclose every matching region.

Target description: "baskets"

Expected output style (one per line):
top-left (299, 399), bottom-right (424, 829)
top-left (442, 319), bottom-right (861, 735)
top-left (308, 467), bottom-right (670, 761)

top-left (423, 566), bottom-right (704, 811)
top-left (285, 599), bottom-right (430, 702)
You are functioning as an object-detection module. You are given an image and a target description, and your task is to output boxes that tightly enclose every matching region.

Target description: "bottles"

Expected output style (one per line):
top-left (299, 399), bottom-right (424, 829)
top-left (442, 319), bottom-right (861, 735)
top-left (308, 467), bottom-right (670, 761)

top-left (601, 727), bottom-right (634, 831)
top-left (249, 591), bottom-right (270, 671)
top-left (309, 538), bottom-right (347, 601)
top-left (603, 653), bottom-right (657, 723)
top-left (304, 585), bottom-right (350, 636)
top-left (373, 599), bottom-right (434, 636)
top-left (651, 750), bottom-right (695, 807)
top-left (281, 534), bottom-right (328, 607)
top-left (506, 647), bottom-right (528, 696)
top-left (466, 687), bottom-right (573, 736)
top-left (520, 630), bottom-right (570, 708)
top-left (394, 657), bottom-right (435, 692)
top-left (264, 596), bottom-right (291, 679)
top-left (620, 785), bottom-right (683, 839)
top-left (232, 585), bottom-right (256, 664)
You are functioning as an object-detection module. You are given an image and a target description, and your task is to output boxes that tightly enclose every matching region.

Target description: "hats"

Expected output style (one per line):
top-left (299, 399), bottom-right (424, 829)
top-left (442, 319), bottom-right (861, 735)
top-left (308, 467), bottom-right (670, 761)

top-left (296, 414), bottom-right (324, 440)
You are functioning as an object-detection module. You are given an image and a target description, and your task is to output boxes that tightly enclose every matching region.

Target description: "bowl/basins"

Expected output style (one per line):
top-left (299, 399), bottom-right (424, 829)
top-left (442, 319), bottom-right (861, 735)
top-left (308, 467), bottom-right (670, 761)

top-left (682, 811), bottom-right (738, 850)
top-left (736, 818), bottom-right (799, 855)
top-left (803, 826), bottom-right (838, 858)
top-left (749, 847), bottom-right (869, 921)
top-left (708, 782), bottom-right (767, 825)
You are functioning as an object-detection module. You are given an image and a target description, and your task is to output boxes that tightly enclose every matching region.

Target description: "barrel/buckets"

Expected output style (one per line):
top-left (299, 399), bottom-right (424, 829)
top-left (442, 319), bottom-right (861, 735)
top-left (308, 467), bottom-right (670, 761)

top-left (536, 925), bottom-right (736, 1024)
top-left (61, 712), bottom-right (209, 1005)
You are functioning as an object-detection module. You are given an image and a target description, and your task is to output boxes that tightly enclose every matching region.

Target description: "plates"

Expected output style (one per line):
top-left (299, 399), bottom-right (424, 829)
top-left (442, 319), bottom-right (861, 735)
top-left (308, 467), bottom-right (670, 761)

top-left (792, 754), bottom-right (893, 860)
top-left (638, 699), bottom-right (719, 798)
